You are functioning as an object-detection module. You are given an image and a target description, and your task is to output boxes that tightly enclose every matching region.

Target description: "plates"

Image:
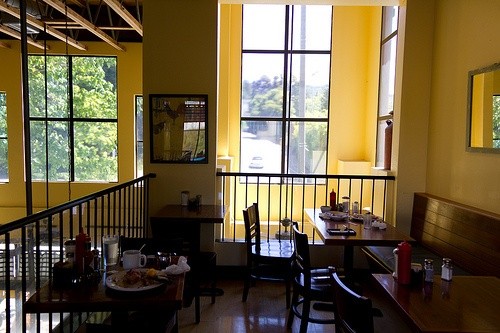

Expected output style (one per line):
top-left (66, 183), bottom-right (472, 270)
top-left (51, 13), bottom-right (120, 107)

top-left (107, 270), bottom-right (165, 291)
top-left (326, 210), bottom-right (348, 221)
top-left (351, 215), bottom-right (379, 220)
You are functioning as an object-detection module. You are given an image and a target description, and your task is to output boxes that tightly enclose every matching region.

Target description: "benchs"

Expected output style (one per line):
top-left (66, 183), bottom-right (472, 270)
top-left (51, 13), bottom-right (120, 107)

top-left (363, 190), bottom-right (500, 276)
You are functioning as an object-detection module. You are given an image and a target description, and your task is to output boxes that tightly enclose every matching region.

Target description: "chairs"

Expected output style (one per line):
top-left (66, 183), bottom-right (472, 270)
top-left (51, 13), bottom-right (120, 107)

top-left (288, 223), bottom-right (374, 333)
top-left (243, 202), bottom-right (295, 308)
top-left (153, 221), bottom-right (215, 323)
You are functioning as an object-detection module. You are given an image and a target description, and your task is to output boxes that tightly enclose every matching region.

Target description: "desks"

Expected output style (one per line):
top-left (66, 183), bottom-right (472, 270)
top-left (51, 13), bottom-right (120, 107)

top-left (25, 256), bottom-right (184, 333)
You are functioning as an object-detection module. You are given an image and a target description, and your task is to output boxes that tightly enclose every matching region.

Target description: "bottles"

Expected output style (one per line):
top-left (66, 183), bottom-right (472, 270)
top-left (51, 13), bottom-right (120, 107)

top-left (424, 258), bottom-right (435, 282)
top-left (396, 236), bottom-right (412, 284)
top-left (338, 196), bottom-right (350, 213)
top-left (363, 211), bottom-right (372, 229)
top-left (441, 257), bottom-right (453, 282)
top-left (64, 227), bottom-right (102, 291)
top-left (352, 201), bottom-right (359, 214)
top-left (180, 190), bottom-right (202, 211)
top-left (392, 247), bottom-right (399, 281)
top-left (330, 188), bottom-right (337, 211)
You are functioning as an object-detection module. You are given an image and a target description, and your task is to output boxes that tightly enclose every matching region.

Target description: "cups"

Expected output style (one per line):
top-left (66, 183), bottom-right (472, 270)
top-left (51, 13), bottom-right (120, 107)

top-left (102, 235), bottom-right (120, 266)
top-left (156, 252), bottom-right (171, 268)
top-left (123, 250), bottom-right (147, 269)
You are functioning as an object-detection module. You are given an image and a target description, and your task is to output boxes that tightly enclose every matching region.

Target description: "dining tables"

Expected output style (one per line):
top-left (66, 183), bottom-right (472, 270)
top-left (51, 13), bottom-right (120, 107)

top-left (306, 209), bottom-right (417, 316)
top-left (150, 204), bottom-right (224, 295)
top-left (372, 274), bottom-right (500, 333)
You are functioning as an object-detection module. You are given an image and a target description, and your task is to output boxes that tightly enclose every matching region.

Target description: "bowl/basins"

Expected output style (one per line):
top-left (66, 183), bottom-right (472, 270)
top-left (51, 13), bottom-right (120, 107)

top-left (320, 206), bottom-right (331, 213)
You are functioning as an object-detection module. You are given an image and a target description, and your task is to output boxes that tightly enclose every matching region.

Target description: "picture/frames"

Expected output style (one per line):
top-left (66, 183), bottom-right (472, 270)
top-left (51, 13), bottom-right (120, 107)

top-left (149, 94), bottom-right (208, 164)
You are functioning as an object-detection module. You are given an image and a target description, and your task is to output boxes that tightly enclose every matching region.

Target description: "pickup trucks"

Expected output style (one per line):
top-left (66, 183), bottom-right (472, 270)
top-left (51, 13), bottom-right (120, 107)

top-left (249, 157), bottom-right (264, 168)
